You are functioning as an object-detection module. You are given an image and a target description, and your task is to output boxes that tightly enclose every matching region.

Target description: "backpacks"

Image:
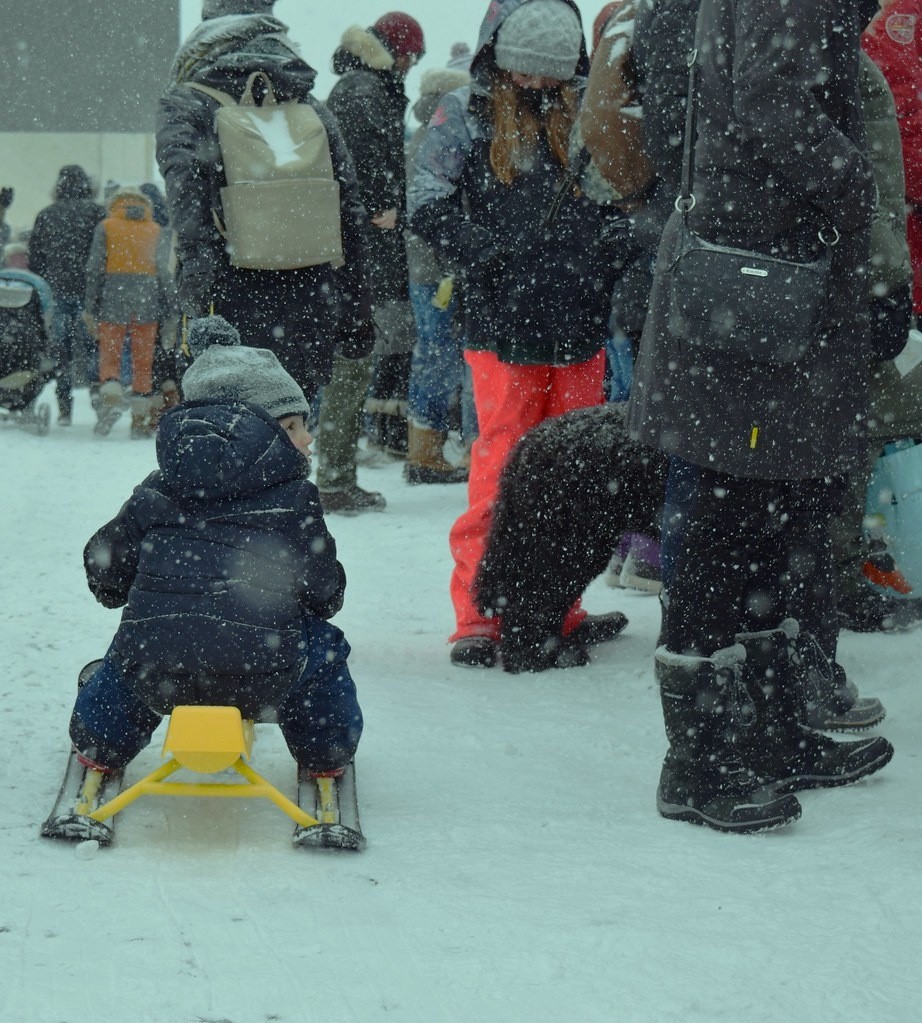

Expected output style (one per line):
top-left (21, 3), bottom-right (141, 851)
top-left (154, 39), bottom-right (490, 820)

top-left (182, 71), bottom-right (347, 271)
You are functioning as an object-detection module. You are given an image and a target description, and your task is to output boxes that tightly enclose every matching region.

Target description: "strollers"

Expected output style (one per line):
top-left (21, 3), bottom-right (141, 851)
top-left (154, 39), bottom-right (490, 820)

top-left (0, 266), bottom-right (68, 439)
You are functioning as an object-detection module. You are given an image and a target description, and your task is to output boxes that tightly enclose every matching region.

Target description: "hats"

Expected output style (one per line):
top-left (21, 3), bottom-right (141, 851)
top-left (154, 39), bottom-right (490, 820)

top-left (374, 12), bottom-right (425, 54)
top-left (181, 315), bottom-right (310, 421)
top-left (494, 0), bottom-right (583, 79)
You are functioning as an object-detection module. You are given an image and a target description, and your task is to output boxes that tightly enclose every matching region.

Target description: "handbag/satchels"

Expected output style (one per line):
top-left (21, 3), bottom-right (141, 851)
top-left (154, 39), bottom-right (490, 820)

top-left (668, 217), bottom-right (837, 366)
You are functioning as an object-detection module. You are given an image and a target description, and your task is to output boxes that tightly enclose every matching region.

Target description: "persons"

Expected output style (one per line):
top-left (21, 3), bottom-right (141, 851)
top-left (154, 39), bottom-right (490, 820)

top-left (85, 185), bottom-right (170, 440)
top-left (313, 12), bottom-right (421, 514)
top-left (0, 0), bottom-right (922, 835)
top-left (71, 313), bottom-right (363, 775)
top-left (30, 166), bottom-right (103, 426)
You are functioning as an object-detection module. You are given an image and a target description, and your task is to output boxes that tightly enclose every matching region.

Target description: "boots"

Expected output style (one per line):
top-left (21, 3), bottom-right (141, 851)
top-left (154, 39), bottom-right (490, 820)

top-left (401, 416), bottom-right (468, 483)
top-left (653, 647), bottom-right (803, 830)
top-left (93, 377), bottom-right (129, 435)
top-left (736, 619), bottom-right (898, 791)
top-left (131, 398), bottom-right (153, 438)
top-left (57, 398), bottom-right (73, 428)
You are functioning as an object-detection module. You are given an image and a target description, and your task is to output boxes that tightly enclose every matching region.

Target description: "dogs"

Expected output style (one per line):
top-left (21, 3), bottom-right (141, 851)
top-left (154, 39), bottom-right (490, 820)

top-left (470, 400), bottom-right (669, 676)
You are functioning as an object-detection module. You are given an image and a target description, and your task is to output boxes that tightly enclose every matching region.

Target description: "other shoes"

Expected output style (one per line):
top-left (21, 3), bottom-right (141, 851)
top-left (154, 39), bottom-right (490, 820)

top-left (836, 586), bottom-right (921, 634)
top-left (502, 637), bottom-right (589, 672)
top-left (452, 637), bottom-right (495, 668)
top-left (568, 611), bottom-right (629, 647)
top-left (805, 685), bottom-right (887, 731)
top-left (318, 487), bottom-right (387, 513)
top-left (606, 558), bottom-right (667, 593)
